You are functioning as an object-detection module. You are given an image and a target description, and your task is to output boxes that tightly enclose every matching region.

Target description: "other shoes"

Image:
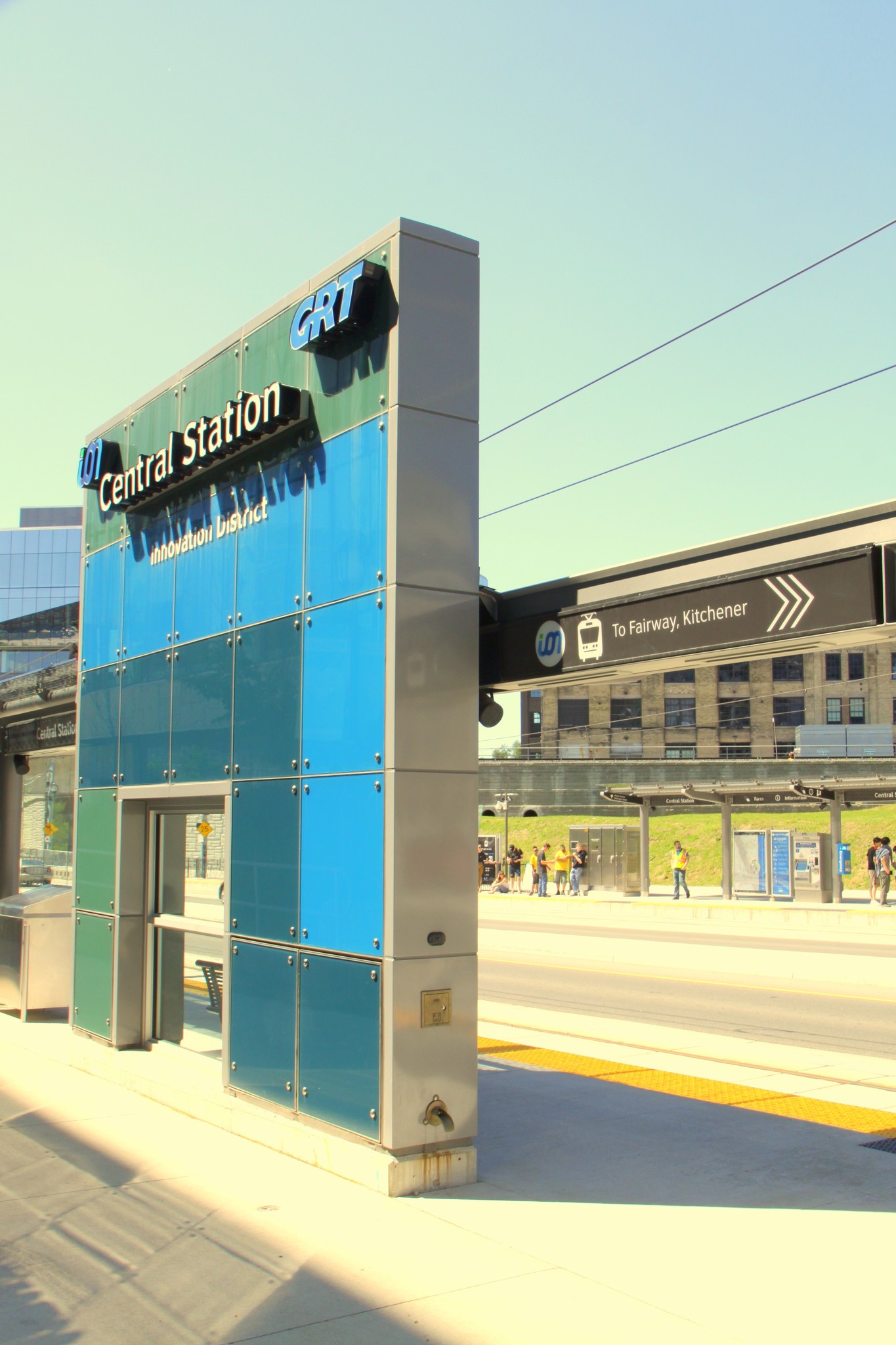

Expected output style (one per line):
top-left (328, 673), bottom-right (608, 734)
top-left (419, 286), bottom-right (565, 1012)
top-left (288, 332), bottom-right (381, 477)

top-left (561, 891), bottom-right (565, 895)
top-left (686, 891), bottom-right (690, 898)
top-left (881, 903), bottom-right (891, 907)
top-left (529, 892), bottom-right (533, 896)
top-left (672, 897), bottom-right (679, 899)
top-left (870, 900), bottom-right (880, 905)
top-left (543, 894), bottom-right (551, 897)
top-left (538, 894), bottom-right (542, 897)
top-left (570, 892), bottom-right (578, 896)
top-left (511, 891), bottom-right (514, 893)
top-left (518, 891), bottom-right (521, 893)
top-left (555, 891), bottom-right (560, 895)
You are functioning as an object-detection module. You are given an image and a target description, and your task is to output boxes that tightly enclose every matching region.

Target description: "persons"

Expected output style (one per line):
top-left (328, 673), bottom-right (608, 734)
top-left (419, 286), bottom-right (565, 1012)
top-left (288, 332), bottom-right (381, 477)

top-left (529, 842), bottom-right (555, 897)
top-left (867, 837), bottom-right (896, 907)
top-left (506, 844), bottom-right (524, 894)
top-left (488, 871), bottom-right (509, 894)
top-left (671, 841), bottom-right (690, 900)
top-left (570, 843), bottom-right (586, 896)
top-left (554, 845), bottom-right (571, 895)
top-left (478, 844), bottom-right (496, 893)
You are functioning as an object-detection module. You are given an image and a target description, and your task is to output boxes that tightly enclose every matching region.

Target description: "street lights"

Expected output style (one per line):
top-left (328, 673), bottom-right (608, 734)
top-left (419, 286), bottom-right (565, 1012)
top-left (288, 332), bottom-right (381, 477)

top-left (495, 793), bottom-right (519, 879)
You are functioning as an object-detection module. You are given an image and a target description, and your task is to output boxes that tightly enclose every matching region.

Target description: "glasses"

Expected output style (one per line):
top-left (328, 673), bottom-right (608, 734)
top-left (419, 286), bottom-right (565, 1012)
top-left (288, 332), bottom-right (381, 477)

top-left (535, 850), bottom-right (539, 851)
top-left (676, 844), bottom-right (681, 846)
top-left (548, 848), bottom-right (549, 850)
top-left (479, 847), bottom-right (482, 848)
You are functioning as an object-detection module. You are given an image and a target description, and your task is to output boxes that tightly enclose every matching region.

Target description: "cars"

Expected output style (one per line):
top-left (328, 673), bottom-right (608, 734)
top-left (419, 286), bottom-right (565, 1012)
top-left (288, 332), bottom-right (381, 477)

top-left (20, 858), bottom-right (53, 886)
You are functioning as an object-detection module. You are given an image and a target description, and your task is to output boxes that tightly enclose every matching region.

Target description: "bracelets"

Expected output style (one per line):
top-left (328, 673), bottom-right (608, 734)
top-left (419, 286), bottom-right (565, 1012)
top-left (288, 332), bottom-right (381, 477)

top-left (518, 859), bottom-right (520, 861)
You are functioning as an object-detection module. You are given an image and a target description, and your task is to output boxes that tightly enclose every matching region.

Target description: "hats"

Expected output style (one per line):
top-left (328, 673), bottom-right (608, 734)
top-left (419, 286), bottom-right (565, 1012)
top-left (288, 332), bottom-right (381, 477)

top-left (509, 844), bottom-right (514, 852)
